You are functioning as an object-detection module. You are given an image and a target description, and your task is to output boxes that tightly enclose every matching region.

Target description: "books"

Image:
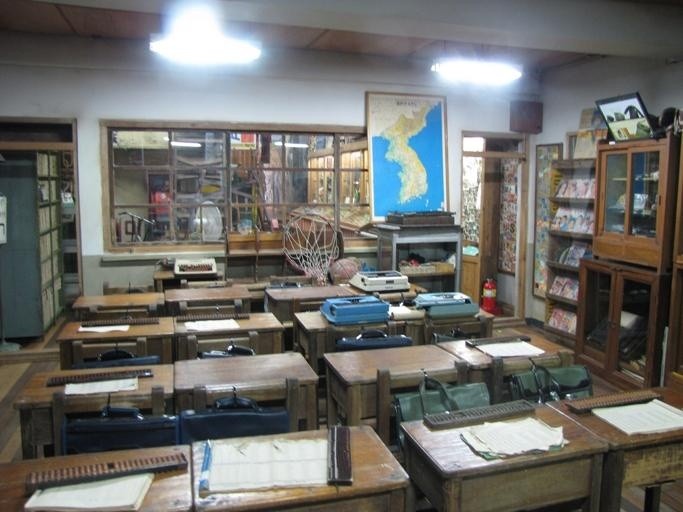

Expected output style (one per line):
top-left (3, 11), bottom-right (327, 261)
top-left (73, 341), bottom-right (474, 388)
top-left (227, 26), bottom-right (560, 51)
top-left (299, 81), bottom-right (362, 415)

top-left (79, 324), bottom-right (129, 332)
top-left (559, 241), bottom-right (586, 267)
top-left (548, 274), bottom-right (578, 301)
top-left (554, 177), bottom-right (596, 199)
top-left (388, 305), bottom-right (425, 320)
top-left (478, 339), bottom-right (545, 358)
top-left (26, 472), bottom-right (153, 511)
top-left (463, 417), bottom-right (570, 460)
top-left (64, 376), bottom-right (138, 395)
top-left (593, 399), bottom-right (683, 436)
top-left (548, 308), bottom-right (576, 334)
top-left (550, 207), bottom-right (594, 233)
top-left (199, 438), bottom-right (332, 494)
top-left (186, 319), bottom-right (240, 333)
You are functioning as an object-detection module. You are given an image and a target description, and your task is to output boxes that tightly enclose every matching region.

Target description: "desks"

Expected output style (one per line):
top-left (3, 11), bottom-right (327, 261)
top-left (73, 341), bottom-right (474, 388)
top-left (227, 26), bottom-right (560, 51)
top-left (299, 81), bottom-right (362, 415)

top-left (0, 442), bottom-right (191, 512)
top-left (173, 351), bottom-right (321, 430)
top-left (152, 262), bottom-right (226, 290)
top-left (56, 314), bottom-right (176, 368)
top-left (13, 363), bottom-right (172, 457)
top-left (72, 290), bottom-right (160, 324)
top-left (163, 285), bottom-right (253, 312)
top-left (436, 333), bottom-right (576, 396)
top-left (546, 386), bottom-right (683, 512)
top-left (263, 283), bottom-right (355, 315)
top-left (397, 404), bottom-right (611, 511)
top-left (408, 297), bottom-right (498, 341)
top-left (193, 423), bottom-right (413, 512)
top-left (342, 281), bottom-right (431, 303)
top-left (320, 342), bottom-right (469, 419)
top-left (175, 308), bottom-right (287, 358)
top-left (294, 308), bottom-right (408, 362)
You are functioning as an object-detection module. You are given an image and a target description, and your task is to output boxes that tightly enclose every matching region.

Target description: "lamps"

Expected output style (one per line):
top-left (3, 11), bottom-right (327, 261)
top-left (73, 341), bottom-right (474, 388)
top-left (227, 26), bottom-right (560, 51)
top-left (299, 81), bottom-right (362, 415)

top-left (273, 142), bottom-right (310, 148)
top-left (170, 141), bottom-right (201, 147)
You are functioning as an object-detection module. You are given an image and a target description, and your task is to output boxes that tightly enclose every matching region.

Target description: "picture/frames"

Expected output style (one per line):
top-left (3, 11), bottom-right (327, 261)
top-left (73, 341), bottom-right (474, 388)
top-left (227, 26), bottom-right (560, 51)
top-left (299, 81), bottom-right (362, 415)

top-left (364, 92), bottom-right (452, 222)
top-left (594, 91), bottom-right (656, 143)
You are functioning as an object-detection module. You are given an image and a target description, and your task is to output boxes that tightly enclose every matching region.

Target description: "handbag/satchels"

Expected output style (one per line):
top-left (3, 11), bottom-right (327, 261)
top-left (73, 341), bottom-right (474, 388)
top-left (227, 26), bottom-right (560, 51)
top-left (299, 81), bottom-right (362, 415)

top-left (429, 328), bottom-right (471, 346)
top-left (71, 349), bottom-right (162, 371)
top-left (263, 282), bottom-right (310, 311)
top-left (393, 376), bottom-right (490, 467)
top-left (62, 407), bottom-right (178, 454)
top-left (336, 328), bottom-right (413, 352)
top-left (178, 396), bottom-right (289, 444)
top-left (510, 363), bottom-right (593, 404)
top-left (195, 345), bottom-right (254, 359)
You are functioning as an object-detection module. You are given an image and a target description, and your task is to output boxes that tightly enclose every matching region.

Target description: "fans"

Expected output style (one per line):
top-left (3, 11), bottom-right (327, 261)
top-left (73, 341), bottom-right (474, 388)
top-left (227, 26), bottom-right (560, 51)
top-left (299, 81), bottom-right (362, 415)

top-left (191, 200), bottom-right (225, 243)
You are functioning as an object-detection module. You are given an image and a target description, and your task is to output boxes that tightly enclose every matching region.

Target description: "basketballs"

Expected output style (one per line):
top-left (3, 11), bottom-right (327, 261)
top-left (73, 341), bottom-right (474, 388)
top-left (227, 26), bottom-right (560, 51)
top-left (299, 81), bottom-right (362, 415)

top-left (330, 259), bottom-right (359, 285)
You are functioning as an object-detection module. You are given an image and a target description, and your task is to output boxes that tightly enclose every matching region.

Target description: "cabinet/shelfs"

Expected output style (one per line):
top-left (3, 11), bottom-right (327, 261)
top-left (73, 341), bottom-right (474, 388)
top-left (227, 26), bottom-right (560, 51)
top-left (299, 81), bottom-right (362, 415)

top-left (572, 256), bottom-right (664, 388)
top-left (590, 139), bottom-right (676, 268)
top-left (546, 158), bottom-right (598, 347)
top-left (0, 153), bottom-right (63, 342)
